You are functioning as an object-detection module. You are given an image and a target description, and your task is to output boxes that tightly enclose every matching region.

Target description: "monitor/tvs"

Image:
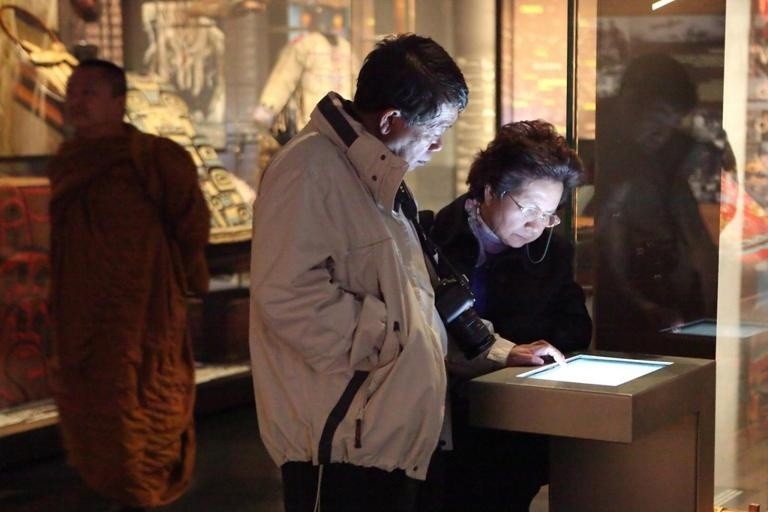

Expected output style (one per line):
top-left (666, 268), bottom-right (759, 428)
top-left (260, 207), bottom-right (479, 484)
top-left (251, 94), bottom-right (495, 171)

top-left (662, 318), bottom-right (768, 339)
top-left (516, 351), bottom-right (674, 388)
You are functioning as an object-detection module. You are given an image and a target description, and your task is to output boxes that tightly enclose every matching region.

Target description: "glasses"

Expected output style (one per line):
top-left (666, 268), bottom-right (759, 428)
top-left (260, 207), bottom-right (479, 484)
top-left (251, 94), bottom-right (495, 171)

top-left (504, 191), bottom-right (561, 228)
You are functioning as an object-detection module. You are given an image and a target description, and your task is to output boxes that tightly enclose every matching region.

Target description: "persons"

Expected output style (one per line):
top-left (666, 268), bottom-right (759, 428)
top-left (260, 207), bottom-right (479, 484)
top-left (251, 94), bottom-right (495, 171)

top-left (432, 120), bottom-right (591, 511)
top-left (247, 34), bottom-right (566, 511)
top-left (252, 11), bottom-right (360, 128)
top-left (598, 48), bottom-right (738, 350)
top-left (43, 59), bottom-right (213, 512)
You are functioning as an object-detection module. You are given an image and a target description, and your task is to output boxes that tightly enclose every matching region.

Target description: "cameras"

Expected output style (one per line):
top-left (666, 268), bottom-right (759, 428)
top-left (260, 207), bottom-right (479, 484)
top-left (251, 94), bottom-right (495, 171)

top-left (435, 274), bottom-right (495, 361)
